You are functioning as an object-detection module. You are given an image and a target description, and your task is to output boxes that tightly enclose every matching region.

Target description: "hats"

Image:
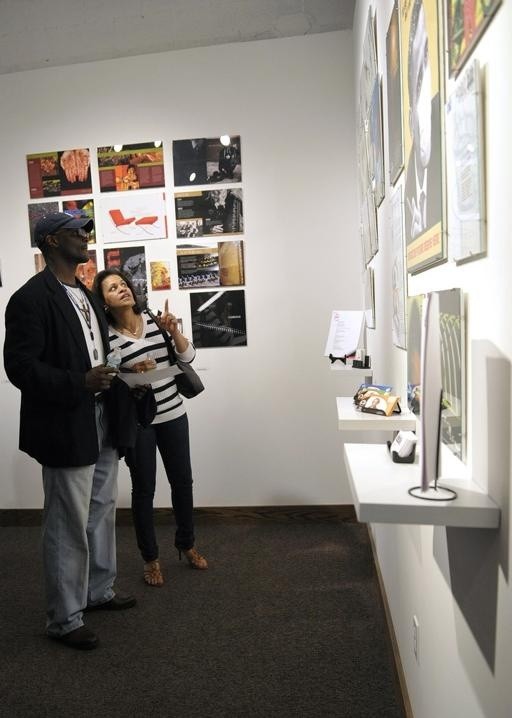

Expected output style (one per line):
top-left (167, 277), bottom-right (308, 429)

top-left (34, 213), bottom-right (94, 240)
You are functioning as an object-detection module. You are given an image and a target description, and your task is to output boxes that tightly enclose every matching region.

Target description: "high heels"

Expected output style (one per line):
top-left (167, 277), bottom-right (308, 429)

top-left (143, 558), bottom-right (163, 585)
top-left (178, 549), bottom-right (208, 568)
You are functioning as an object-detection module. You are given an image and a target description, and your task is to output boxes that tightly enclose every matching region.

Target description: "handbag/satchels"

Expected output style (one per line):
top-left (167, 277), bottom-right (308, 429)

top-left (172, 362), bottom-right (205, 399)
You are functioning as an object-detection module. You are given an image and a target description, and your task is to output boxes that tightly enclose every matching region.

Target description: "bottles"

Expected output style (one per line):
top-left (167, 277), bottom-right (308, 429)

top-left (146, 352), bottom-right (156, 365)
top-left (106, 347), bottom-right (122, 375)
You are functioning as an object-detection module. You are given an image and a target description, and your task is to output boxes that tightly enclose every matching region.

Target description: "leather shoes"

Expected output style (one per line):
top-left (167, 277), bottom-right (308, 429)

top-left (103, 595), bottom-right (137, 608)
top-left (49, 627), bottom-right (98, 650)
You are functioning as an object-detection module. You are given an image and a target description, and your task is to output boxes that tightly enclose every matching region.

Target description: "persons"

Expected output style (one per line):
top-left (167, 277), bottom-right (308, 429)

top-left (404, 0), bottom-right (442, 245)
top-left (4, 212), bottom-right (122, 648)
top-left (122, 165), bottom-right (139, 189)
top-left (91, 269), bottom-right (208, 584)
